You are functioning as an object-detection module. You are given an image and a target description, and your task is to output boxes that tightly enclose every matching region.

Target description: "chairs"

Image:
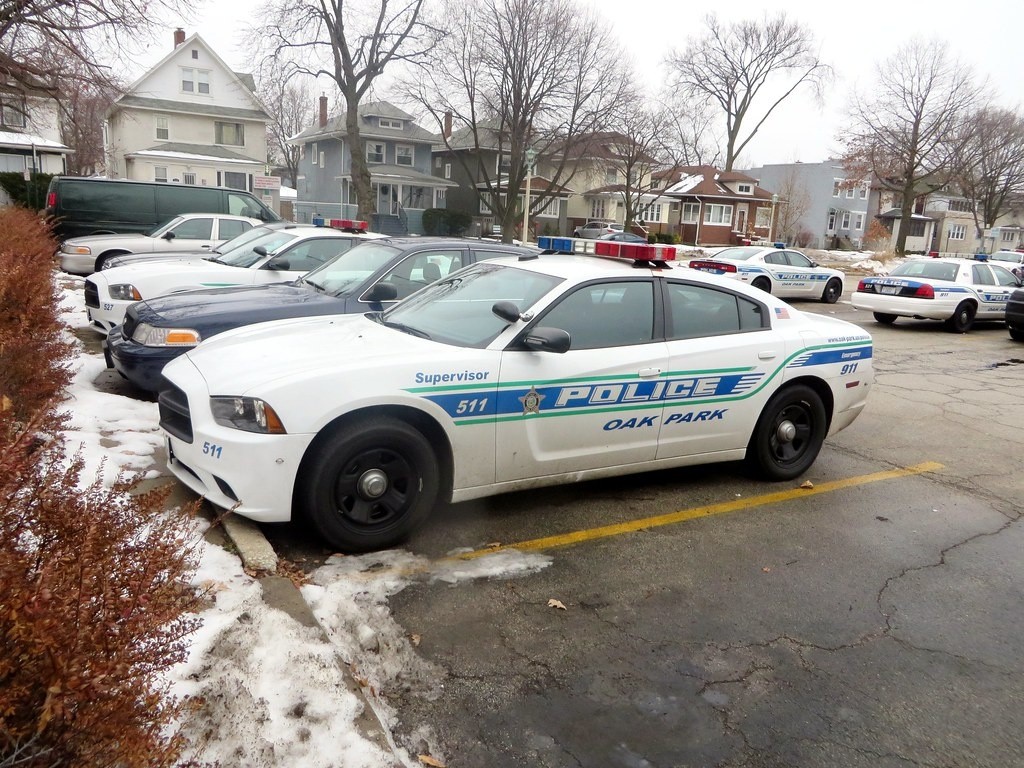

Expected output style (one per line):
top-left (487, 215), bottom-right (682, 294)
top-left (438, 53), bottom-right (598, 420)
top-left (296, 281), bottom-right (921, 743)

top-left (607, 288), bottom-right (651, 344)
top-left (414, 263), bottom-right (441, 289)
top-left (390, 258), bottom-right (416, 283)
top-left (304, 244), bottom-right (321, 268)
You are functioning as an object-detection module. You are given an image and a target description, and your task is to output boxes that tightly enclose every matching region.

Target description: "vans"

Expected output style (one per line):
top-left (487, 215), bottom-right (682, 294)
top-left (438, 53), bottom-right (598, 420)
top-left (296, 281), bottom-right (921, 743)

top-left (44, 174), bottom-right (293, 251)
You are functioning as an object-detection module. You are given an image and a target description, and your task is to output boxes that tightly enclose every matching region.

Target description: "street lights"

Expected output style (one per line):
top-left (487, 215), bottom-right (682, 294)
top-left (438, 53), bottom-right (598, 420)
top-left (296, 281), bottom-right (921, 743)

top-left (768, 194), bottom-right (779, 243)
top-left (523, 144), bottom-right (535, 245)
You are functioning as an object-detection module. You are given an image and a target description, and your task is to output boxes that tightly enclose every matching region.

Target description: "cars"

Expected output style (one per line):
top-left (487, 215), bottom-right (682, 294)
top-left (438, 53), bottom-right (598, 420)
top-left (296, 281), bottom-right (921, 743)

top-left (572, 221), bottom-right (624, 239)
top-left (1005, 279), bottom-right (1024, 343)
top-left (687, 240), bottom-right (845, 306)
top-left (594, 232), bottom-right (648, 245)
top-left (986, 247), bottom-right (1024, 282)
top-left (102, 220), bottom-right (317, 272)
top-left (56, 212), bottom-right (262, 274)
top-left (100, 236), bottom-right (544, 393)
top-left (83, 215), bottom-right (458, 336)
top-left (156, 232), bottom-right (873, 555)
top-left (851, 250), bottom-right (1024, 333)
